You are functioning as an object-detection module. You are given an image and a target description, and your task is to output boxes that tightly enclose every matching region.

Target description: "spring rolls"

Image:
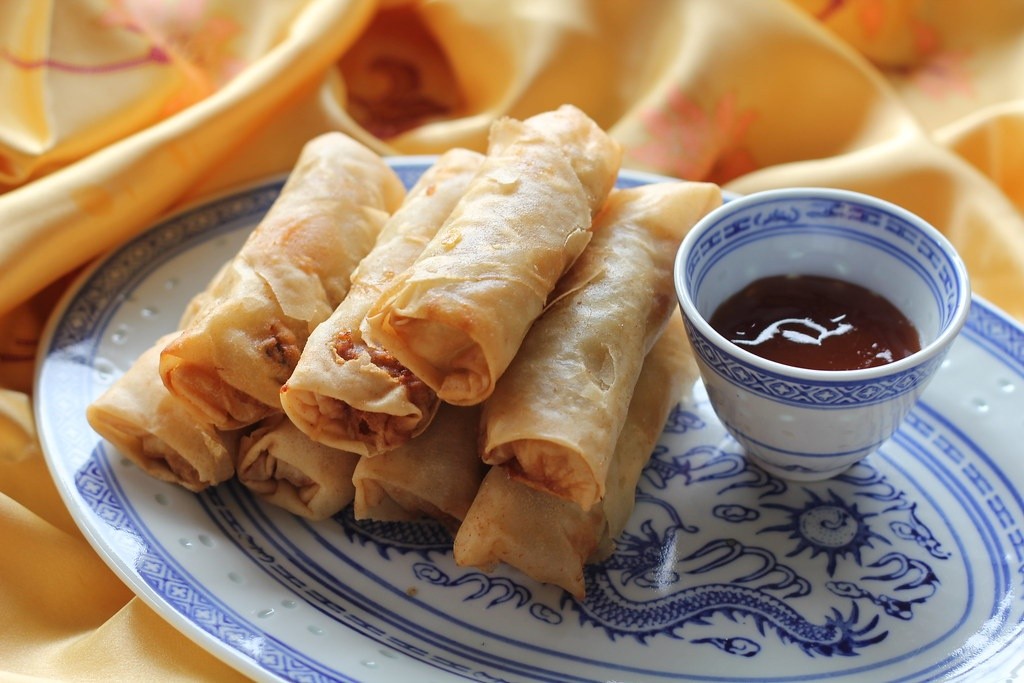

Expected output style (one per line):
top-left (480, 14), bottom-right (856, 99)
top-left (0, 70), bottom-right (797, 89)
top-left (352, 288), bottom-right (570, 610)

top-left (81, 102), bottom-right (712, 604)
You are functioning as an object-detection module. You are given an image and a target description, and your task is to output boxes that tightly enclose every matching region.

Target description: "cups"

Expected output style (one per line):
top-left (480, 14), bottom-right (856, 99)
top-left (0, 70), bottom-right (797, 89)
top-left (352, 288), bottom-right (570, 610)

top-left (674, 183), bottom-right (973, 482)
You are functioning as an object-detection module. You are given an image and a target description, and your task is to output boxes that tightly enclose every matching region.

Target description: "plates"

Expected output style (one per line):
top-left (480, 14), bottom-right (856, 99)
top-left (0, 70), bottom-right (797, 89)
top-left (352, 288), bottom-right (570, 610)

top-left (30, 153), bottom-right (1024, 683)
